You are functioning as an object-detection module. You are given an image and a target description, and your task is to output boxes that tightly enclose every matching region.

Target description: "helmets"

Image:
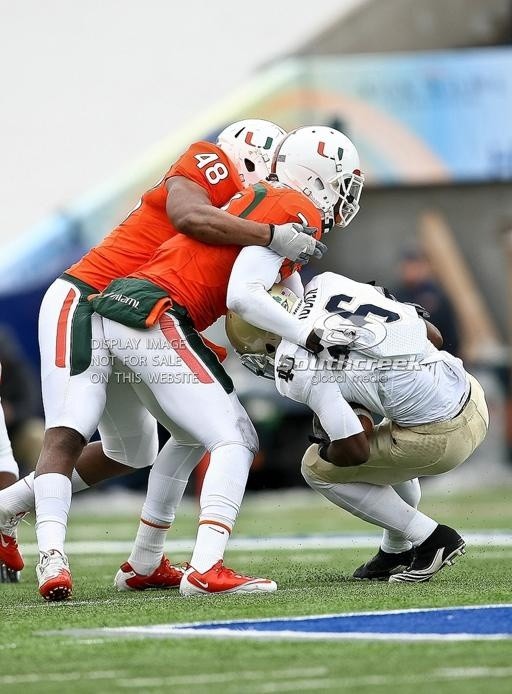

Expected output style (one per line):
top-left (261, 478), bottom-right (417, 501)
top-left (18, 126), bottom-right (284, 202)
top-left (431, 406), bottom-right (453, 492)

top-left (225, 282), bottom-right (300, 365)
top-left (275, 123), bottom-right (363, 219)
top-left (216, 117), bottom-right (287, 189)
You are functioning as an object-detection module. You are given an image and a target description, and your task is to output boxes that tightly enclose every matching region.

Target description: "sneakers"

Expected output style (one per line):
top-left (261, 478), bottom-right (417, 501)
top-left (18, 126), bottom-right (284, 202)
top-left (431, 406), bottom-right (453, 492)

top-left (0, 507), bottom-right (31, 571)
top-left (114, 553), bottom-right (188, 591)
top-left (352, 543), bottom-right (415, 581)
top-left (177, 558), bottom-right (278, 597)
top-left (35, 548), bottom-right (76, 602)
top-left (388, 523), bottom-right (467, 583)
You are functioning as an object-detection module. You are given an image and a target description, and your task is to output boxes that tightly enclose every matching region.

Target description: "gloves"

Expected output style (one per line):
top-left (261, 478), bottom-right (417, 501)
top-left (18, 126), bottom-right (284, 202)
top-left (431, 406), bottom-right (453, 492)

top-left (366, 281), bottom-right (432, 320)
top-left (268, 221), bottom-right (329, 266)
top-left (307, 326), bottom-right (384, 369)
top-left (310, 435), bottom-right (331, 463)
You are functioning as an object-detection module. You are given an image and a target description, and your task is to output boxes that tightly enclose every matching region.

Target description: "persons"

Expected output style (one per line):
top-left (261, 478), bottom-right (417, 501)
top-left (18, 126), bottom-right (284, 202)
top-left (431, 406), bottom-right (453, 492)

top-left (226, 271), bottom-right (489, 584)
top-left (1, 118), bottom-right (366, 602)
top-left (388, 247), bottom-right (460, 356)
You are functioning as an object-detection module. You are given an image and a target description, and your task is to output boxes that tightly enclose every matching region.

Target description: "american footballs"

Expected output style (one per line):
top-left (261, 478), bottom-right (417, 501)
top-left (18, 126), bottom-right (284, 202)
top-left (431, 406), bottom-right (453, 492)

top-left (349, 400), bottom-right (374, 440)
